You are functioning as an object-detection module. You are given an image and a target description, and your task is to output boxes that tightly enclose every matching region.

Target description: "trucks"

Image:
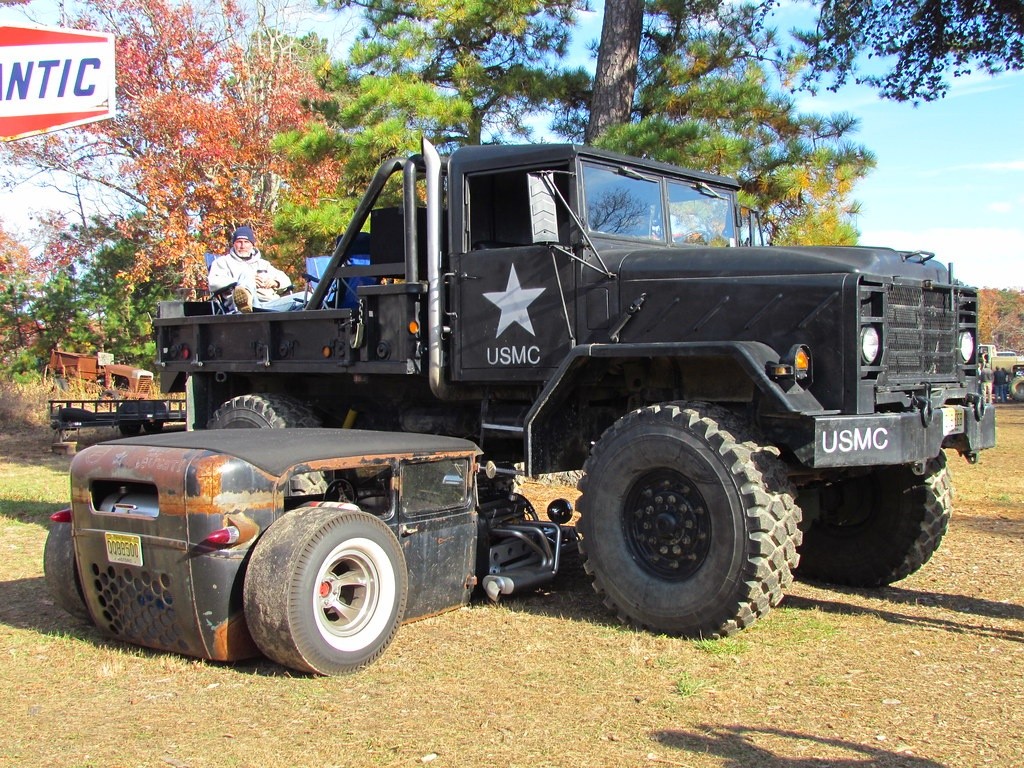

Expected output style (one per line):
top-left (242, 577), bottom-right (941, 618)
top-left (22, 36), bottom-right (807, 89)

top-left (977, 343), bottom-right (1024, 402)
top-left (151, 133), bottom-right (997, 642)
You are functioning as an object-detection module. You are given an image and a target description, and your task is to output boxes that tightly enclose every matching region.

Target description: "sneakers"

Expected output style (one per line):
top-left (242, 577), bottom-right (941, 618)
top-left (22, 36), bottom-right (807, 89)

top-left (233, 285), bottom-right (253, 313)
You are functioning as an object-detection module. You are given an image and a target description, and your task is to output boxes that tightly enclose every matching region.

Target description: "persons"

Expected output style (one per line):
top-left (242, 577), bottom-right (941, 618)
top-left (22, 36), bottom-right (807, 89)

top-left (207, 226), bottom-right (305, 312)
top-left (978, 363), bottom-right (1009, 403)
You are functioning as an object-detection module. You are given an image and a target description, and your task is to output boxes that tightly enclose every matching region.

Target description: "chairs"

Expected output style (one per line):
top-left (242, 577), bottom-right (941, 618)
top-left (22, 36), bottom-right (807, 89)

top-left (204, 232), bottom-right (372, 309)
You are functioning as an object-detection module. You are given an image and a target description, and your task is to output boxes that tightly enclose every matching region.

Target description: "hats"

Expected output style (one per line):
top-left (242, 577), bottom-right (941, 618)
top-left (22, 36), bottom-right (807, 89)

top-left (233, 226), bottom-right (254, 246)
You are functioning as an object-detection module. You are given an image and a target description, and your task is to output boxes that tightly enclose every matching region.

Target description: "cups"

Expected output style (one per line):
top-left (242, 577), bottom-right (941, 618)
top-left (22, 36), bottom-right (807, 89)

top-left (257, 269), bottom-right (267, 288)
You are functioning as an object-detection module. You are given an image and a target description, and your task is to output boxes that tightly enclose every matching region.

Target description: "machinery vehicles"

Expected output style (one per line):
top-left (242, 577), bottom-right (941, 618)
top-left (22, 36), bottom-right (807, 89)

top-left (44, 350), bottom-right (155, 412)
